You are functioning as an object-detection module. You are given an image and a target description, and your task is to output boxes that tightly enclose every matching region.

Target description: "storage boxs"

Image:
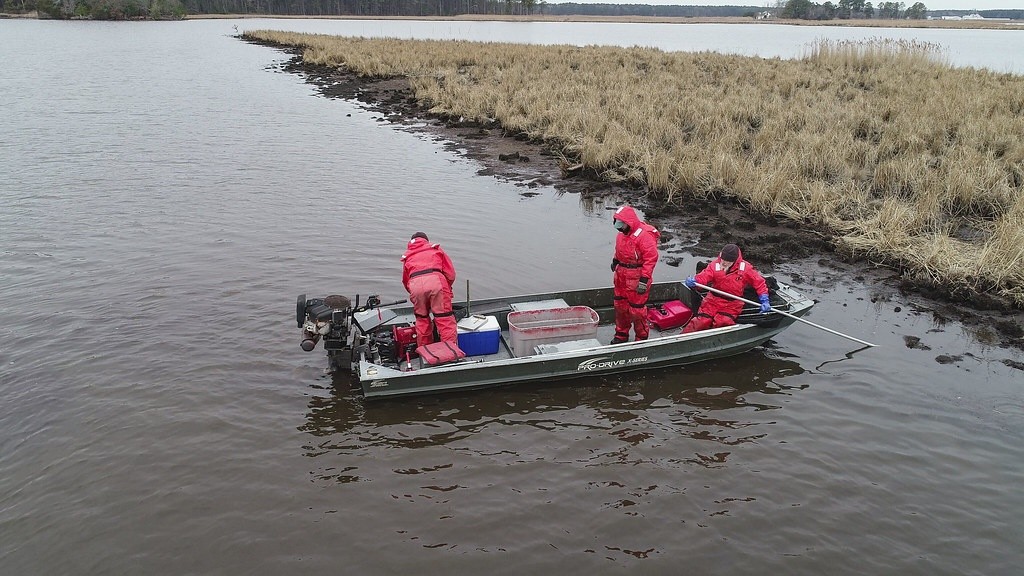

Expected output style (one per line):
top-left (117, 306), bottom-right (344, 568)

top-left (419, 355), bottom-right (463, 370)
top-left (507, 306), bottom-right (600, 357)
top-left (508, 298), bottom-right (570, 312)
top-left (532, 338), bottom-right (602, 355)
top-left (456, 316), bottom-right (501, 357)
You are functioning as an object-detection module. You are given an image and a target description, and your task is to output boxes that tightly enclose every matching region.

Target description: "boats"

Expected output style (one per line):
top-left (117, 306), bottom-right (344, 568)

top-left (293, 265), bottom-right (818, 402)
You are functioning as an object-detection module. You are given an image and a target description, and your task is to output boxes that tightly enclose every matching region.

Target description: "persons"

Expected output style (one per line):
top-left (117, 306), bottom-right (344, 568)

top-left (400, 230), bottom-right (459, 352)
top-left (680, 243), bottom-right (771, 334)
top-left (611, 205), bottom-right (659, 344)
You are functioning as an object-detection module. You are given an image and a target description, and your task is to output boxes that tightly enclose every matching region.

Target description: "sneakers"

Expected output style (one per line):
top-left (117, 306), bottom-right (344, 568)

top-left (610, 337), bottom-right (628, 345)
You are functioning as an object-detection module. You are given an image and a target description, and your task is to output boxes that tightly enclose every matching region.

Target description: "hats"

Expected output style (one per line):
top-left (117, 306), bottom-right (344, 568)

top-left (614, 218), bottom-right (625, 228)
top-left (720, 244), bottom-right (739, 262)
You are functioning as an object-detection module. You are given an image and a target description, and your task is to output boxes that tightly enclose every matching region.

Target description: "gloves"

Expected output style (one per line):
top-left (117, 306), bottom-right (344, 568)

top-left (610, 258), bottom-right (618, 272)
top-left (636, 277), bottom-right (648, 294)
top-left (759, 294), bottom-right (771, 313)
top-left (685, 276), bottom-right (696, 288)
